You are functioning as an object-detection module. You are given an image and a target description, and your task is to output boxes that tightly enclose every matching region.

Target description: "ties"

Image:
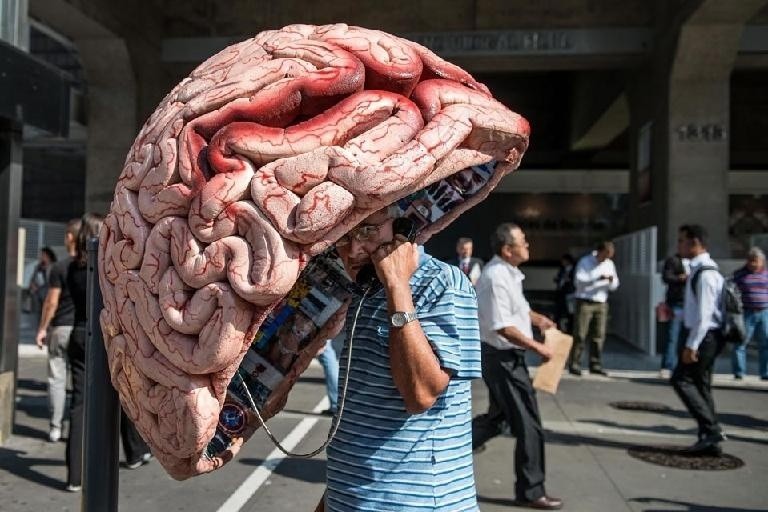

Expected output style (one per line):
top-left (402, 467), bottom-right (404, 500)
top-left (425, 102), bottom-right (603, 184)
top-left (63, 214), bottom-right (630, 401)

top-left (463, 261), bottom-right (468, 277)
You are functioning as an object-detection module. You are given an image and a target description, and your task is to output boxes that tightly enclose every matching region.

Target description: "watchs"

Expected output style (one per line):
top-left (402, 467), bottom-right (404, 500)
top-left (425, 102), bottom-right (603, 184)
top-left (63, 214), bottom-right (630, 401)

top-left (387, 310), bottom-right (418, 328)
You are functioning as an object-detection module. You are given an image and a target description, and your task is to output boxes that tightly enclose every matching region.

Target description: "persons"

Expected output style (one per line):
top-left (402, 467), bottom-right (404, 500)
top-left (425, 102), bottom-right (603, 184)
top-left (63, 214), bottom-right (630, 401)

top-left (556, 255), bottom-right (573, 334)
top-left (315, 205), bottom-right (482, 512)
top-left (668, 225), bottom-right (728, 459)
top-left (733, 248), bottom-right (767, 381)
top-left (569, 242), bottom-right (620, 376)
top-left (315, 341), bottom-right (341, 416)
top-left (445, 166), bottom-right (488, 195)
top-left (661, 255), bottom-right (689, 378)
top-left (471, 224), bottom-right (563, 510)
top-left (270, 310), bottom-right (318, 369)
top-left (449, 235), bottom-right (482, 284)
top-left (29, 213), bottom-right (151, 491)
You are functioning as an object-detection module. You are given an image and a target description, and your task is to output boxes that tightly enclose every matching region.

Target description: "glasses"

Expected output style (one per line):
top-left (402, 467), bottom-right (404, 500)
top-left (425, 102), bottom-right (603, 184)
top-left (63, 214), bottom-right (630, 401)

top-left (336, 216), bottom-right (393, 248)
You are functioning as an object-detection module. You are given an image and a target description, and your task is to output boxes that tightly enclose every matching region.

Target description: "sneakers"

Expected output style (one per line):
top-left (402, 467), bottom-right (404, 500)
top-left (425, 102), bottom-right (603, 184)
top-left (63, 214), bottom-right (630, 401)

top-left (658, 369), bottom-right (672, 381)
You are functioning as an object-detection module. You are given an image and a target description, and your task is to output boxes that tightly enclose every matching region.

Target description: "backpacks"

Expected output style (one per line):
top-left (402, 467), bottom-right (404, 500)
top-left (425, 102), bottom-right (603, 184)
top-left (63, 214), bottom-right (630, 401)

top-left (690, 267), bottom-right (747, 349)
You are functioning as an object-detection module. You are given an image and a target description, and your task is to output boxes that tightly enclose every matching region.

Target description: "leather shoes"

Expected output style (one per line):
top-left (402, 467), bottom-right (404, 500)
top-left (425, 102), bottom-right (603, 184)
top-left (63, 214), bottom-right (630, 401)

top-left (568, 368), bottom-right (582, 377)
top-left (678, 443), bottom-right (724, 459)
top-left (697, 430), bottom-right (730, 443)
top-left (518, 491), bottom-right (563, 510)
top-left (318, 407), bottom-right (336, 414)
top-left (589, 369), bottom-right (608, 378)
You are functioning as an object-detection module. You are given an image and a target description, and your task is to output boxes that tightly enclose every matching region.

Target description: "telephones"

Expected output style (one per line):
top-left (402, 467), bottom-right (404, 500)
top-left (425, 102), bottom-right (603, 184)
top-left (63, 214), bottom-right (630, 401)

top-left (355, 217), bottom-right (417, 299)
